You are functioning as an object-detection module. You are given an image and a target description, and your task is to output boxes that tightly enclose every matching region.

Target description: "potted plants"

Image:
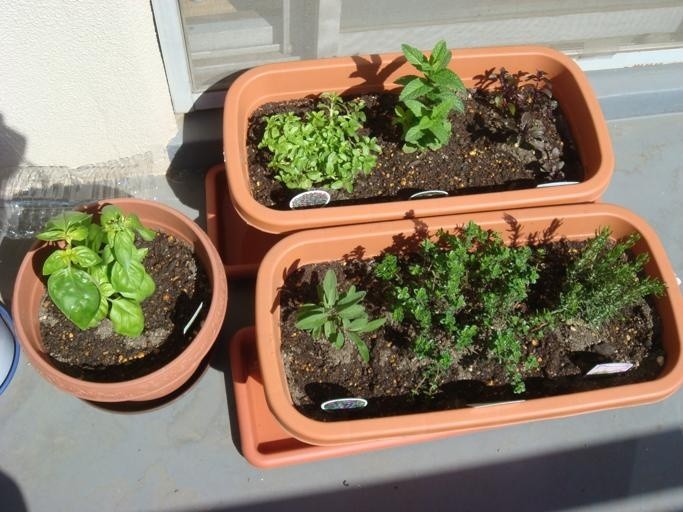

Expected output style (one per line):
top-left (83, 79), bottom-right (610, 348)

top-left (225, 203), bottom-right (682, 470)
top-left (9, 198), bottom-right (228, 415)
top-left (203, 43), bottom-right (614, 280)
